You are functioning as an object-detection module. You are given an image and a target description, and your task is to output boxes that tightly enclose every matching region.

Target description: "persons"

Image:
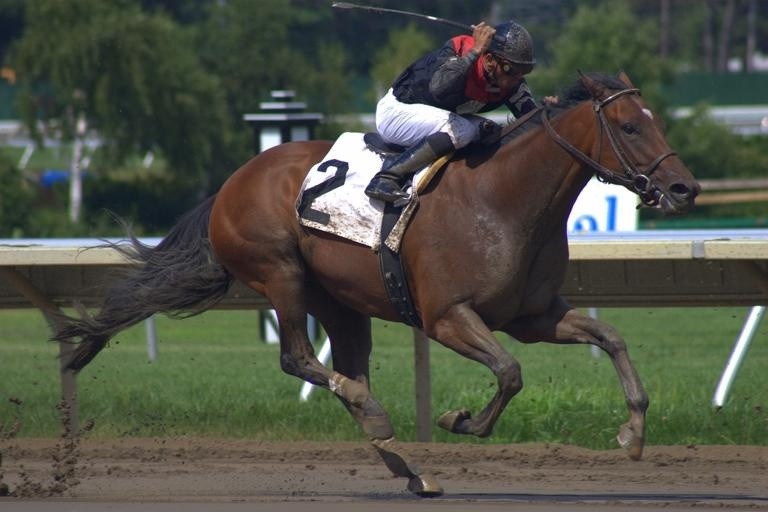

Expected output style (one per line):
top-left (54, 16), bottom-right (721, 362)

top-left (364, 22), bottom-right (558, 204)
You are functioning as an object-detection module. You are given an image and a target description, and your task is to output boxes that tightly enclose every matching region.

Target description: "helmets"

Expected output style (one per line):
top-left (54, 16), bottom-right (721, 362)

top-left (491, 20), bottom-right (536, 65)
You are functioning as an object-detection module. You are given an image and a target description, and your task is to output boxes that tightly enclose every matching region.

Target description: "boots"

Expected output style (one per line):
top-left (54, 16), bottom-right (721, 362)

top-left (365, 136), bottom-right (437, 202)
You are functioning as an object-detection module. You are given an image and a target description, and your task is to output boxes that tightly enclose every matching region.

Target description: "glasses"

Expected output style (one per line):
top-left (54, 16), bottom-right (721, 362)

top-left (497, 58), bottom-right (532, 75)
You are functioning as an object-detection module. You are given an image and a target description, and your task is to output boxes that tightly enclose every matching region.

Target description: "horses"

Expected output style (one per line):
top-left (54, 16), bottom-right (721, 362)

top-left (46, 67), bottom-right (702, 497)
